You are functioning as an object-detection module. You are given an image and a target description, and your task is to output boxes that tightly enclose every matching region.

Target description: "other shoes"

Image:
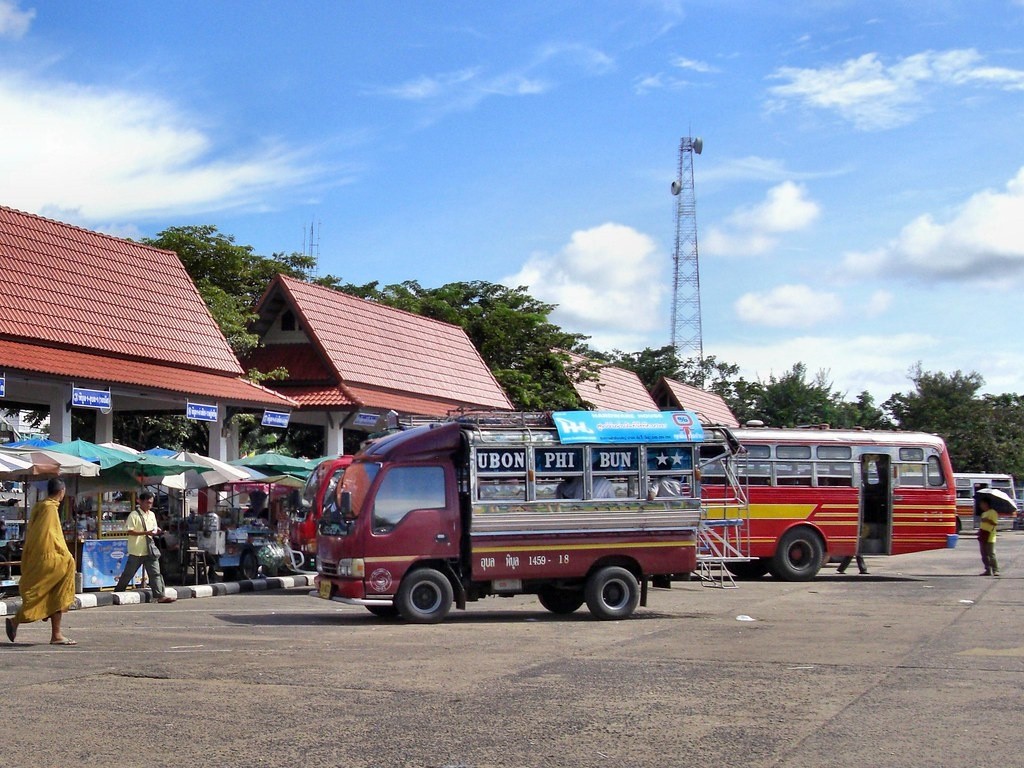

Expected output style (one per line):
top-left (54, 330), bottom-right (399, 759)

top-left (859, 572), bottom-right (870, 574)
top-left (837, 569), bottom-right (845, 574)
top-left (994, 573), bottom-right (999, 576)
top-left (980, 572), bottom-right (991, 576)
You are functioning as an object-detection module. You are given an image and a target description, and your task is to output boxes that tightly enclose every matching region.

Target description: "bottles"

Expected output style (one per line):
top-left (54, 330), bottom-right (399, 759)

top-left (87, 515), bottom-right (98, 531)
top-left (102, 500), bottom-right (131, 512)
top-left (5, 524), bottom-right (11, 539)
top-left (15, 524), bottom-right (19, 539)
top-left (101, 519), bottom-right (127, 532)
top-left (242, 517), bottom-right (264, 527)
top-left (11, 524), bottom-right (15, 539)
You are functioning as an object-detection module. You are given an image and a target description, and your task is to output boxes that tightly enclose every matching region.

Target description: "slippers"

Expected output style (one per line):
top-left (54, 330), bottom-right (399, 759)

top-left (158, 598), bottom-right (176, 603)
top-left (50, 638), bottom-right (77, 645)
top-left (6, 618), bottom-right (16, 642)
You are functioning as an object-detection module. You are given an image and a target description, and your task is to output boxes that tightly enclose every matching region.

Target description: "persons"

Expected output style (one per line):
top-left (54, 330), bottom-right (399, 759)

top-left (114, 491), bottom-right (177, 603)
top-left (557, 455), bottom-right (683, 501)
top-left (837, 555), bottom-right (869, 574)
top-left (6, 477), bottom-right (77, 647)
top-left (977, 498), bottom-right (1000, 576)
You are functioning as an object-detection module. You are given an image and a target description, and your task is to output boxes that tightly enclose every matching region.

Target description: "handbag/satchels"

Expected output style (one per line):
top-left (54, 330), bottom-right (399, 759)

top-left (978, 529), bottom-right (990, 543)
top-left (146, 536), bottom-right (161, 561)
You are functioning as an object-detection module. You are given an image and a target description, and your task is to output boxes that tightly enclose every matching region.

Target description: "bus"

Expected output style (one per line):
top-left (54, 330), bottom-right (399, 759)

top-left (289, 424), bottom-right (960, 582)
top-left (899, 471), bottom-right (1018, 535)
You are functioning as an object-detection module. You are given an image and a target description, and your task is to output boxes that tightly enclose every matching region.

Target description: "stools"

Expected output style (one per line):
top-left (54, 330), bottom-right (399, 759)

top-left (182, 550), bottom-right (210, 585)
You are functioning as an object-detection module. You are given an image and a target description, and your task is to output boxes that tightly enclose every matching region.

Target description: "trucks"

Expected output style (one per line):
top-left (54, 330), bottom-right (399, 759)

top-left (307, 409), bottom-right (706, 624)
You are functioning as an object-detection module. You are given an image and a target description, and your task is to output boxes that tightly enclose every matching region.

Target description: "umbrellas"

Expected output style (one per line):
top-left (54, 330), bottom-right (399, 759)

top-left (0, 437), bottom-right (347, 587)
top-left (974, 487), bottom-right (1018, 514)
